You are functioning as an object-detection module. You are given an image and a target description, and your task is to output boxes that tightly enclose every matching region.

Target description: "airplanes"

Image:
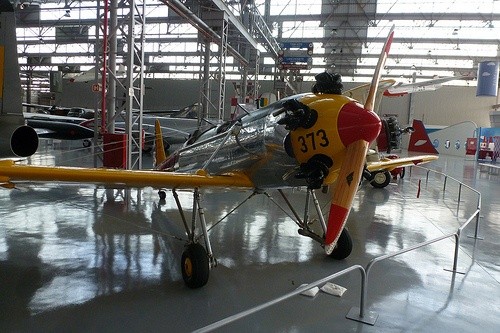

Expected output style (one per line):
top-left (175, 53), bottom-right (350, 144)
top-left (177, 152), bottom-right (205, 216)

top-left (0, 1), bottom-right (468, 289)
